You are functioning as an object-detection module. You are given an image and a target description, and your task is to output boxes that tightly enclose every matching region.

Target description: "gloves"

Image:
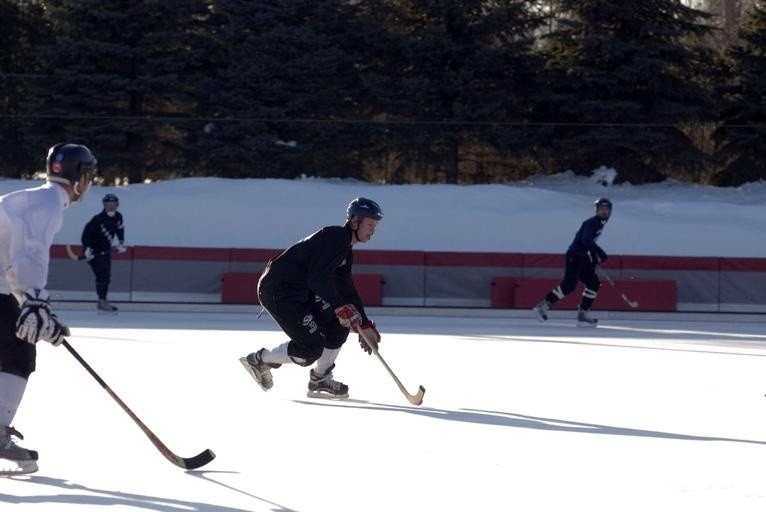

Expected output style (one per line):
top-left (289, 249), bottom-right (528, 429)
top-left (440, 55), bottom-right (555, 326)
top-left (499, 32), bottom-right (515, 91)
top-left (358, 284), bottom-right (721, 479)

top-left (356, 319), bottom-right (381, 355)
top-left (333, 302), bottom-right (364, 332)
top-left (586, 247), bottom-right (598, 268)
top-left (596, 245), bottom-right (609, 264)
top-left (83, 247), bottom-right (94, 260)
top-left (115, 242), bottom-right (127, 255)
top-left (12, 288), bottom-right (51, 345)
top-left (43, 311), bottom-right (72, 347)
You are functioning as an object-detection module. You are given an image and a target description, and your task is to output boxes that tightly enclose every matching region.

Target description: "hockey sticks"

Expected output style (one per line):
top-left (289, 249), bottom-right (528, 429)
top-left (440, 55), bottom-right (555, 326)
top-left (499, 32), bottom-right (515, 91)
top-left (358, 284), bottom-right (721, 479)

top-left (356, 325), bottom-right (425, 405)
top-left (596, 264), bottom-right (639, 308)
top-left (66, 244), bottom-right (118, 261)
top-left (62, 338), bottom-right (216, 469)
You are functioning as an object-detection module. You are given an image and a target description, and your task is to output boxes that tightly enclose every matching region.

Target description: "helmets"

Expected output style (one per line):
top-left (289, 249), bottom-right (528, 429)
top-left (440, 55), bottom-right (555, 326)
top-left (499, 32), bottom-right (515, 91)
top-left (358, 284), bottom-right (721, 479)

top-left (594, 197), bottom-right (612, 208)
top-left (345, 196), bottom-right (384, 221)
top-left (44, 141), bottom-right (100, 187)
top-left (101, 192), bottom-right (119, 203)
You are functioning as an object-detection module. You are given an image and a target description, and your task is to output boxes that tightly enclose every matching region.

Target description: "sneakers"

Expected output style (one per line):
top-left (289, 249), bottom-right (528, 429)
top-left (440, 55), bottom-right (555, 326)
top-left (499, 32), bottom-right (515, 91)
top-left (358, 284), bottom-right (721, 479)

top-left (97, 298), bottom-right (118, 312)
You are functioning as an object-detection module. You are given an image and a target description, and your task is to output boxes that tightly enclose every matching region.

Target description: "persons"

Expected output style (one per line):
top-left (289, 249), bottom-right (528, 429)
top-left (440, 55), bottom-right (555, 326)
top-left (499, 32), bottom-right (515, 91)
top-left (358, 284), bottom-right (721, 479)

top-left (0, 141), bottom-right (99, 461)
top-left (81, 193), bottom-right (128, 311)
top-left (246, 196), bottom-right (380, 396)
top-left (536, 197), bottom-right (613, 324)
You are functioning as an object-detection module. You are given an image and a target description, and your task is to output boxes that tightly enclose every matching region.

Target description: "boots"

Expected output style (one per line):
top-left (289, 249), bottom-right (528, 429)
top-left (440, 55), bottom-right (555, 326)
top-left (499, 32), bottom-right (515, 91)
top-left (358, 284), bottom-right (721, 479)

top-left (306, 362), bottom-right (350, 402)
top-left (575, 304), bottom-right (600, 327)
top-left (0, 425), bottom-right (39, 477)
top-left (241, 348), bottom-right (283, 390)
top-left (533, 298), bottom-right (552, 322)
